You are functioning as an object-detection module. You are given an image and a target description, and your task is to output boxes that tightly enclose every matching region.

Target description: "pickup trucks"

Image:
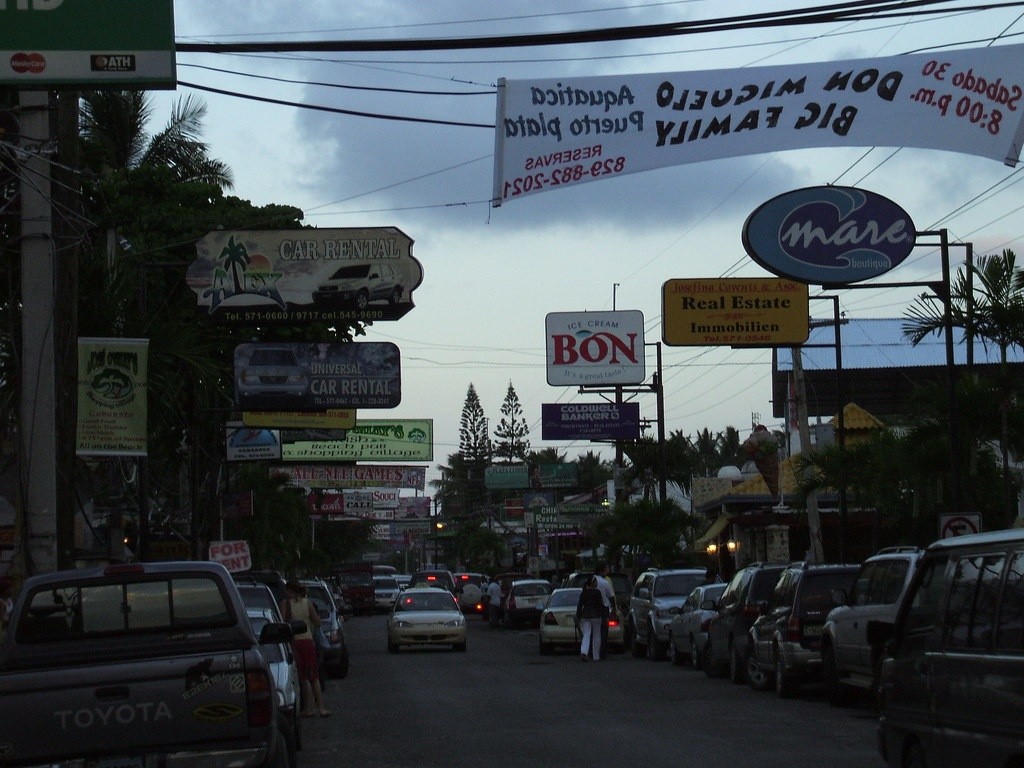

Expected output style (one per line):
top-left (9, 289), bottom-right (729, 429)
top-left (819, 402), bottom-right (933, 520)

top-left (0, 560), bottom-right (295, 768)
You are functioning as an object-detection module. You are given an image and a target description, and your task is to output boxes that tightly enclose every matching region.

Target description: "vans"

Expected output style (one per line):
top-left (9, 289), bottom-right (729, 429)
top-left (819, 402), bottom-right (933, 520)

top-left (878, 525), bottom-right (1024, 768)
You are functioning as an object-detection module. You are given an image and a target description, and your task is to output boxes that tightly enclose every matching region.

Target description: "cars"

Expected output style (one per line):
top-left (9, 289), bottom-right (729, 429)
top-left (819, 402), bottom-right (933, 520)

top-left (388, 586), bottom-right (468, 656)
top-left (668, 582), bottom-right (729, 671)
top-left (536, 586), bottom-right (626, 656)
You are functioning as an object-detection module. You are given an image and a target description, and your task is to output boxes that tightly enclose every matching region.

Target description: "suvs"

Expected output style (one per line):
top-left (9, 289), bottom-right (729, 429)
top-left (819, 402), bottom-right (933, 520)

top-left (403, 567), bottom-right (463, 598)
top-left (504, 578), bottom-right (552, 630)
top-left (565, 569), bottom-right (634, 608)
top-left (743, 560), bottom-right (863, 699)
top-left (820, 543), bottom-right (927, 713)
top-left (627, 565), bottom-right (723, 662)
top-left (700, 561), bottom-right (791, 686)
top-left (163, 564), bottom-right (411, 754)
top-left (454, 572), bottom-right (485, 613)
top-left (491, 572), bottom-right (533, 620)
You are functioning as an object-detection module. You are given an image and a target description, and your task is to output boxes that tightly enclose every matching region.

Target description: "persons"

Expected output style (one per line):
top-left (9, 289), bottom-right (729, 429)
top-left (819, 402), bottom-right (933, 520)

top-left (480, 574), bottom-right (502, 630)
top-left (280, 580), bottom-right (332, 718)
top-left (575, 562), bottom-right (617, 662)
top-left (549, 575), bottom-right (561, 591)
top-left (698, 570), bottom-right (717, 586)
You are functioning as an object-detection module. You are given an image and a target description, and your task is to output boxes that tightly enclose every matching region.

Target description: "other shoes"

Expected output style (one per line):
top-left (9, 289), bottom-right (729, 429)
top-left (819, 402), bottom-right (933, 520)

top-left (319, 713), bottom-right (332, 718)
top-left (300, 713), bottom-right (316, 718)
top-left (580, 654), bottom-right (588, 662)
